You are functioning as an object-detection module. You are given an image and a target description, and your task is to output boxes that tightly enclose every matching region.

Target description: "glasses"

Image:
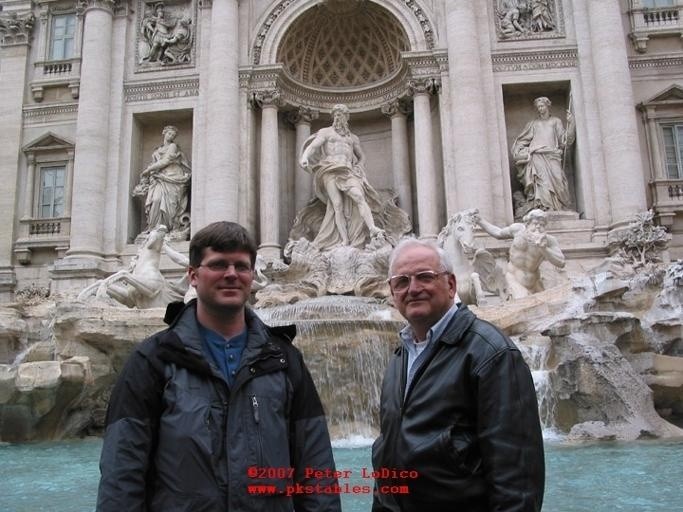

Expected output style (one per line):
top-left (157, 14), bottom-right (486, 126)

top-left (385, 271), bottom-right (448, 293)
top-left (198, 261), bottom-right (253, 273)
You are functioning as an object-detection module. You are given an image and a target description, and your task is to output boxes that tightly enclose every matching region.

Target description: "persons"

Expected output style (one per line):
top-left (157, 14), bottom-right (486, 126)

top-left (473, 208), bottom-right (567, 297)
top-left (94, 222), bottom-right (343, 511)
top-left (368, 237), bottom-right (545, 512)
top-left (512, 97), bottom-right (576, 212)
top-left (301, 102), bottom-right (386, 245)
top-left (135, 6), bottom-right (168, 66)
top-left (501, 2), bottom-right (528, 35)
top-left (134, 125), bottom-right (189, 229)
top-left (531, 0), bottom-right (554, 33)
top-left (163, 15), bottom-right (191, 64)
